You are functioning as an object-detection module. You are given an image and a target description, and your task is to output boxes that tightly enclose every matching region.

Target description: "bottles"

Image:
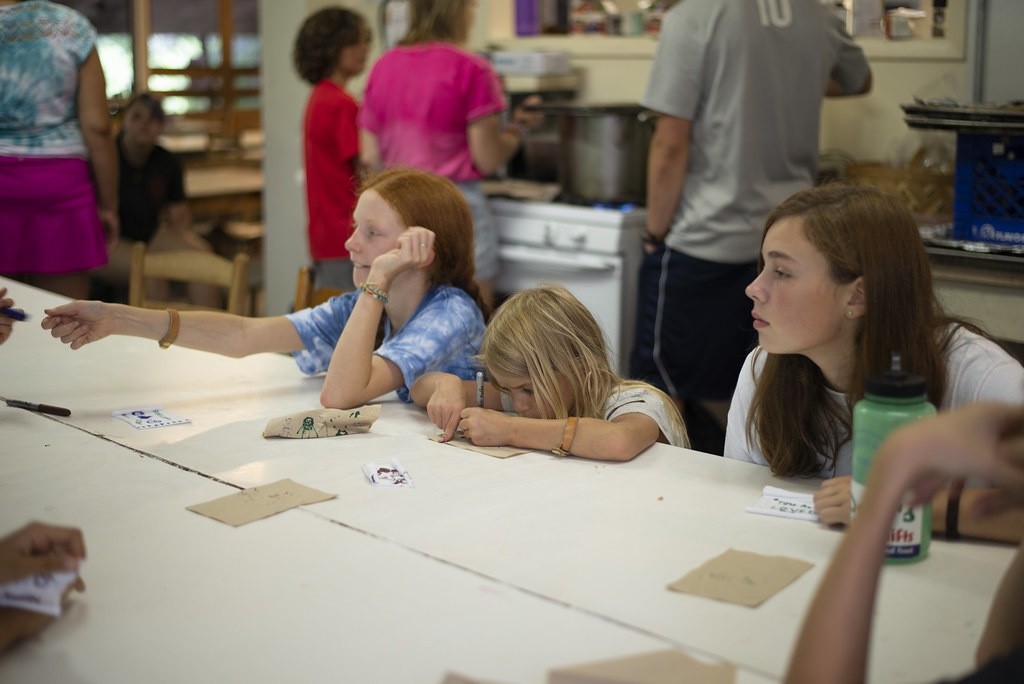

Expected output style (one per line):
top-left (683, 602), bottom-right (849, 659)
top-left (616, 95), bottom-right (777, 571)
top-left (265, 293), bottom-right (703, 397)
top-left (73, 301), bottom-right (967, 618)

top-left (516, 1), bottom-right (537, 35)
top-left (849, 368), bottom-right (939, 565)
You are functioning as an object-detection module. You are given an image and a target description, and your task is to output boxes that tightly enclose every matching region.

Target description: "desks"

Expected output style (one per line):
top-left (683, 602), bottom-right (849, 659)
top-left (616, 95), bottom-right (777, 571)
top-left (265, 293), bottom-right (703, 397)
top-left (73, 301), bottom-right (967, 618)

top-left (182, 163), bottom-right (262, 224)
top-left (0, 257), bottom-right (1024, 684)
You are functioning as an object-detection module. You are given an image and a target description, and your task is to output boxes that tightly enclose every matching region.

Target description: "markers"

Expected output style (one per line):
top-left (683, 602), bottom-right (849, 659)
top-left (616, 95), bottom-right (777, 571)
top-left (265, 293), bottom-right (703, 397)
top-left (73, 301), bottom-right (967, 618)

top-left (476, 372), bottom-right (484, 407)
top-left (5, 399), bottom-right (71, 417)
top-left (0, 307), bottom-right (26, 321)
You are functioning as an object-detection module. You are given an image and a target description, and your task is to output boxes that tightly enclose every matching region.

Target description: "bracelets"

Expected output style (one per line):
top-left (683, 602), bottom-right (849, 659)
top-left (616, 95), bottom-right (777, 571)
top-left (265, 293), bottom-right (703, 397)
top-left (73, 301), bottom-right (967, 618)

top-left (158, 308), bottom-right (180, 349)
top-left (506, 119), bottom-right (535, 140)
top-left (946, 477), bottom-right (965, 539)
top-left (640, 226), bottom-right (671, 243)
top-left (361, 281), bottom-right (389, 304)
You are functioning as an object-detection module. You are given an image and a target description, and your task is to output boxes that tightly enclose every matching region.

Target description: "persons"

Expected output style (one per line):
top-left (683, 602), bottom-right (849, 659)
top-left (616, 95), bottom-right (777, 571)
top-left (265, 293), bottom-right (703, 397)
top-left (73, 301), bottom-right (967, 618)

top-left (0, 0), bottom-right (228, 310)
top-left (629, 0), bottom-right (874, 455)
top-left (0, 288), bottom-right (25, 346)
top-left (291, 0), bottom-right (544, 321)
top-left (723, 181), bottom-right (1024, 543)
top-left (786, 404), bottom-right (1024, 684)
top-left (0, 520), bottom-right (86, 654)
top-left (42, 167), bottom-right (487, 409)
top-left (410, 284), bottom-right (691, 463)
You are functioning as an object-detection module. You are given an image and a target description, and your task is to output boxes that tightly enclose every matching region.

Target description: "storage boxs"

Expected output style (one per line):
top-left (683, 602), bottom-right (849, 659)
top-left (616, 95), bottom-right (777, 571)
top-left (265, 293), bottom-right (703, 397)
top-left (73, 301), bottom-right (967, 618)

top-left (953, 131), bottom-right (1024, 249)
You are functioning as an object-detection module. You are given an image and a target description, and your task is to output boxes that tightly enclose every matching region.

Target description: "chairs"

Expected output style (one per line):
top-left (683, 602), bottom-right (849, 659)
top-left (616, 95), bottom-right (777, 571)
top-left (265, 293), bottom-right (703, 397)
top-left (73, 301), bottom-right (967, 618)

top-left (124, 238), bottom-right (253, 316)
top-left (295, 264), bottom-right (342, 311)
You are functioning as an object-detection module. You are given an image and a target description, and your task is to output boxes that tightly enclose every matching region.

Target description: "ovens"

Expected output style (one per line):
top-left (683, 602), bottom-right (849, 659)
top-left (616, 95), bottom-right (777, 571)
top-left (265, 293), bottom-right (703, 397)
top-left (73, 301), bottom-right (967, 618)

top-left (490, 242), bottom-right (627, 379)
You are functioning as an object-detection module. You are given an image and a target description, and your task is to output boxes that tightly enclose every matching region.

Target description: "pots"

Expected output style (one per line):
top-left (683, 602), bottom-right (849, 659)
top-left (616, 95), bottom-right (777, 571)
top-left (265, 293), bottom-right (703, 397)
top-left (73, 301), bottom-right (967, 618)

top-left (547, 108), bottom-right (656, 204)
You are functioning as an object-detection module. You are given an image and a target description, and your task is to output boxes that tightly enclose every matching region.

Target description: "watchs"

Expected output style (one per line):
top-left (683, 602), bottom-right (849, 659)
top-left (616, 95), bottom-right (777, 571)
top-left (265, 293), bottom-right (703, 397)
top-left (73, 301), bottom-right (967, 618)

top-left (551, 416), bottom-right (579, 457)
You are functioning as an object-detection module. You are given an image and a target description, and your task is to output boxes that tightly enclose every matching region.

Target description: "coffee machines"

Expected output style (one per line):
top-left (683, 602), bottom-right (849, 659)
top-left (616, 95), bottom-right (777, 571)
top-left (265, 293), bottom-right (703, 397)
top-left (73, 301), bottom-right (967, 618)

top-left (501, 69), bottom-right (579, 202)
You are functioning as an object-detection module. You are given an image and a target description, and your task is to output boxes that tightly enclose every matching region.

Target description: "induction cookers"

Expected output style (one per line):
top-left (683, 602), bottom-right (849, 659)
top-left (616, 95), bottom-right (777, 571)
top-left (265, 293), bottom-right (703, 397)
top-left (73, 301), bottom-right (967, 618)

top-left (482, 190), bottom-right (649, 214)
